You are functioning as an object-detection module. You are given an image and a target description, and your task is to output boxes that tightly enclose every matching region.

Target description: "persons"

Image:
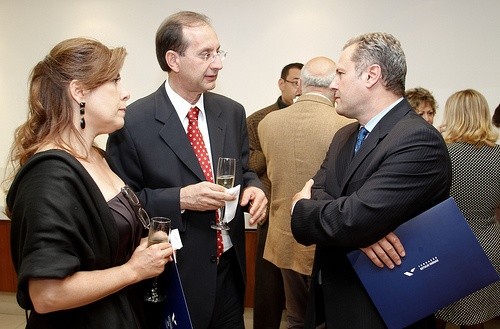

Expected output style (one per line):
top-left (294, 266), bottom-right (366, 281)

top-left (257, 56), bottom-right (357, 329)
top-left (404, 87), bottom-right (438, 125)
top-left (246, 63), bottom-right (306, 329)
top-left (491, 105), bottom-right (500, 128)
top-left (2, 36), bottom-right (173, 329)
top-left (288, 31), bottom-right (452, 329)
top-left (105, 12), bottom-right (272, 329)
top-left (436, 88), bottom-right (500, 329)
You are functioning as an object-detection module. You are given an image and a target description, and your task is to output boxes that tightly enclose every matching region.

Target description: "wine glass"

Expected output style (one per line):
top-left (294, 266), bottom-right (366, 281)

top-left (143, 216), bottom-right (172, 303)
top-left (211, 157), bottom-right (236, 230)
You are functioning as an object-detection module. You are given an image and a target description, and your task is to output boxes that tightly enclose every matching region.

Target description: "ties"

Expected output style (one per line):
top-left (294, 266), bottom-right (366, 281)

top-left (355, 127), bottom-right (368, 154)
top-left (187, 107), bottom-right (223, 257)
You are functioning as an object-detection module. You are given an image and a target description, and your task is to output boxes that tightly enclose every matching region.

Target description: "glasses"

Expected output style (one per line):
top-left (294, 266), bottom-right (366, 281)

top-left (176, 50), bottom-right (227, 65)
top-left (283, 78), bottom-right (299, 88)
